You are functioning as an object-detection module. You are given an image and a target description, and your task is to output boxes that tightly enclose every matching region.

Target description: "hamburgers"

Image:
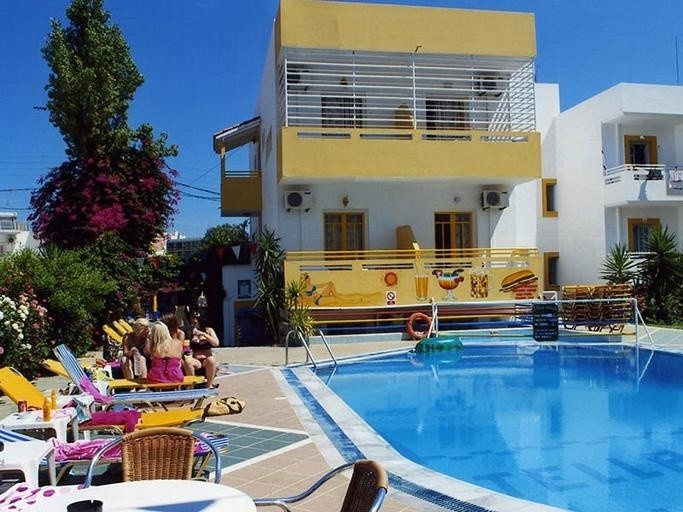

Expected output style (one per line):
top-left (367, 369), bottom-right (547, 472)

top-left (499, 268), bottom-right (538, 294)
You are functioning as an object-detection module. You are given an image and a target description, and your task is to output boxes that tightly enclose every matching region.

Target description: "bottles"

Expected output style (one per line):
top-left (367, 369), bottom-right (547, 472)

top-left (49, 389), bottom-right (57, 409)
top-left (42, 397), bottom-right (51, 421)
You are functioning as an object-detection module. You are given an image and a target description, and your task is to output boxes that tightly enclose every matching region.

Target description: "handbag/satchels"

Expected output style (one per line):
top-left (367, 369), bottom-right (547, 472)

top-left (205, 397), bottom-right (246, 416)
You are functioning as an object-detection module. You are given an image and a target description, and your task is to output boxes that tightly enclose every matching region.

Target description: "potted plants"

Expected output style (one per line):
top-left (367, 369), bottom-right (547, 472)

top-left (197, 224), bottom-right (249, 261)
top-left (249, 224), bottom-right (319, 346)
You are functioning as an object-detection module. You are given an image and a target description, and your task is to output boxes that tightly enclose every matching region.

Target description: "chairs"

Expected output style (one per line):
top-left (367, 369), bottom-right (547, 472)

top-left (560, 283), bottom-right (633, 334)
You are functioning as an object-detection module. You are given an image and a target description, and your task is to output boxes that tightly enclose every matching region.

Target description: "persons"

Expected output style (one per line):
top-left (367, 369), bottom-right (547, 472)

top-left (158, 312), bottom-right (185, 354)
top-left (121, 318), bottom-right (152, 393)
top-left (182, 312), bottom-right (220, 391)
top-left (143, 320), bottom-right (185, 392)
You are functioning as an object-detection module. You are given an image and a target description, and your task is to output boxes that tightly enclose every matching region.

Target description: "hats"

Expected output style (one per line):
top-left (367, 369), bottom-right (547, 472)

top-left (134, 318), bottom-right (149, 326)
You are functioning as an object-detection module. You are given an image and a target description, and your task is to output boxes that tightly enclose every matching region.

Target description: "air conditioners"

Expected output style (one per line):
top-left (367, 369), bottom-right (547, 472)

top-left (478, 75), bottom-right (504, 97)
top-left (481, 190), bottom-right (510, 211)
top-left (280, 63), bottom-right (311, 92)
top-left (284, 190), bottom-right (313, 213)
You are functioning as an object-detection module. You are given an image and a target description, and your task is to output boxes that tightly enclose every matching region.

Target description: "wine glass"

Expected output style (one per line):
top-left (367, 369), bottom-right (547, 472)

top-left (437, 276), bottom-right (461, 301)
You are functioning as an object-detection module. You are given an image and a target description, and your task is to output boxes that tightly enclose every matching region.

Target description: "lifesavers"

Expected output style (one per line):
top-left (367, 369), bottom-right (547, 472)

top-left (407, 313), bottom-right (432, 338)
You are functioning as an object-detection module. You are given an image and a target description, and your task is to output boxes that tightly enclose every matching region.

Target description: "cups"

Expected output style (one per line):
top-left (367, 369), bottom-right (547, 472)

top-left (469, 273), bottom-right (488, 299)
top-left (414, 274), bottom-right (428, 301)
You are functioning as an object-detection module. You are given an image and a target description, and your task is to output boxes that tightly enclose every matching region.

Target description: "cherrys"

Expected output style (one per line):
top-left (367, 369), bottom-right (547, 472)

top-left (454, 275), bottom-right (465, 283)
